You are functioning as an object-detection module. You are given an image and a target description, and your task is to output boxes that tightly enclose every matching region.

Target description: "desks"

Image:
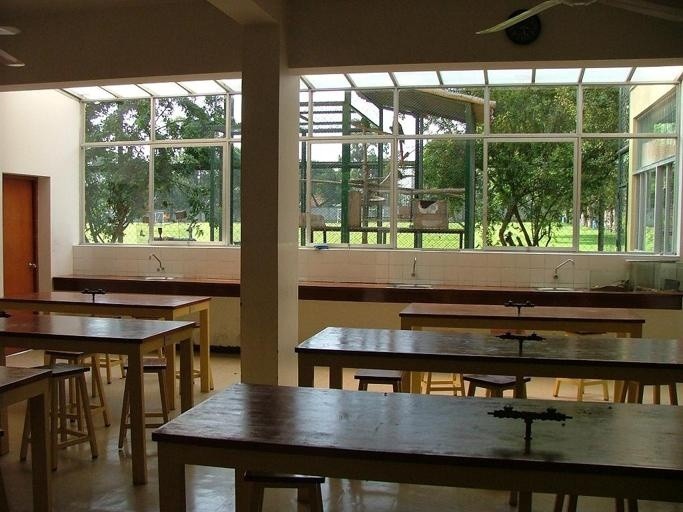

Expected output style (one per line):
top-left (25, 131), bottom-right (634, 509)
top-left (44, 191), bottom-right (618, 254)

top-left (1, 276), bottom-right (682, 511)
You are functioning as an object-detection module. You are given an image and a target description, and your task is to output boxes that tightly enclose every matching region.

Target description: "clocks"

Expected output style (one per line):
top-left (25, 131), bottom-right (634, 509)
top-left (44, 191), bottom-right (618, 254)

top-left (503, 7), bottom-right (542, 47)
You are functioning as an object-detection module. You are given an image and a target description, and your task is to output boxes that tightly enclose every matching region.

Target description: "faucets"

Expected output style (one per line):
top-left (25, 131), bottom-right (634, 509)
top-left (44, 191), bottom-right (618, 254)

top-left (147, 252), bottom-right (165, 272)
top-left (410, 257), bottom-right (417, 277)
top-left (553, 258), bottom-right (576, 280)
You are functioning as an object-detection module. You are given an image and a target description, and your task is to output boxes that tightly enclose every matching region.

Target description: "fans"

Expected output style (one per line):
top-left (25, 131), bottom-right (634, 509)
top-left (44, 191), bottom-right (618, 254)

top-left (0, 25), bottom-right (26, 70)
top-left (471, 0), bottom-right (683, 43)
